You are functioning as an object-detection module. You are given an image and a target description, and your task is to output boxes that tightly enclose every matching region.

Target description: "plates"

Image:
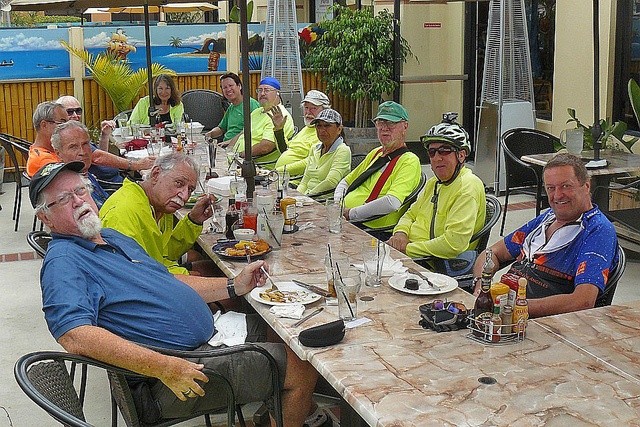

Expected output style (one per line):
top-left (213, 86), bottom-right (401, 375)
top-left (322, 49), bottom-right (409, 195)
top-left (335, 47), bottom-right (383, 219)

top-left (251, 281), bottom-right (321, 306)
top-left (181, 192), bottom-right (221, 209)
top-left (388, 271), bottom-right (459, 295)
top-left (256, 169), bottom-right (279, 182)
top-left (212, 240), bottom-right (272, 260)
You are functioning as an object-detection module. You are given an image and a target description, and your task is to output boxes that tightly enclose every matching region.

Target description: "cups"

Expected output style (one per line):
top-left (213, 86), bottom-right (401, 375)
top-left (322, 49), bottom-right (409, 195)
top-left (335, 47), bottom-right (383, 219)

top-left (208, 138), bottom-right (217, 168)
top-left (181, 141), bottom-right (196, 157)
top-left (147, 143), bottom-right (162, 156)
top-left (264, 211), bottom-right (284, 250)
top-left (361, 240), bottom-right (384, 287)
top-left (227, 152), bottom-right (238, 171)
top-left (333, 270), bottom-right (361, 321)
top-left (229, 177), bottom-right (245, 200)
top-left (122, 126), bottom-right (129, 138)
top-left (118, 118), bottom-right (126, 131)
top-left (275, 170), bottom-right (289, 189)
top-left (324, 252), bottom-right (349, 298)
top-left (240, 195), bottom-right (257, 229)
top-left (326, 195), bottom-right (342, 233)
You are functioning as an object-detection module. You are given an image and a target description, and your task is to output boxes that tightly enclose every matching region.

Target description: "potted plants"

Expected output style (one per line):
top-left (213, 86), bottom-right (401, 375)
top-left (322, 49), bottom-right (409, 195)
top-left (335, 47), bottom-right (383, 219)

top-left (303, 4), bottom-right (421, 153)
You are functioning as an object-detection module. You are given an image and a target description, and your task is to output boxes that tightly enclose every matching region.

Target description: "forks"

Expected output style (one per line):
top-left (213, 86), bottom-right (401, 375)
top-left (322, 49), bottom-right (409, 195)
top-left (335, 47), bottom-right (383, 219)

top-left (260, 266), bottom-right (284, 296)
top-left (209, 201), bottom-right (223, 229)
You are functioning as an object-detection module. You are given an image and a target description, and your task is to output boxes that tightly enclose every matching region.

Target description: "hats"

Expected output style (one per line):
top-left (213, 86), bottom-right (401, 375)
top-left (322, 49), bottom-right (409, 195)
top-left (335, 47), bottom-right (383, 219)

top-left (65, 108), bottom-right (82, 116)
top-left (309, 109), bottom-right (343, 126)
top-left (372, 101), bottom-right (409, 123)
top-left (28, 161), bottom-right (86, 208)
top-left (301, 90), bottom-right (330, 106)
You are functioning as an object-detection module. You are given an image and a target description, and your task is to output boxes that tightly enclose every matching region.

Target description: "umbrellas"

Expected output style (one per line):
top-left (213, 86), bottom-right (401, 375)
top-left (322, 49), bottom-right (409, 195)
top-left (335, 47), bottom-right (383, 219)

top-left (0, 0), bottom-right (219, 124)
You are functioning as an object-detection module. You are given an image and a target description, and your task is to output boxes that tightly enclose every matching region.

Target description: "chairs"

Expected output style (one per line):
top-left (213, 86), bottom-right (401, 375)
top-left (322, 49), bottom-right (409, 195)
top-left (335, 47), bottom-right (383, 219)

top-left (253, 126), bottom-right (298, 162)
top-left (592, 180), bottom-right (640, 254)
top-left (0, 136), bottom-right (29, 231)
top-left (315, 171), bottom-right (427, 229)
top-left (288, 153), bottom-right (368, 199)
top-left (181, 90), bottom-right (223, 132)
top-left (366, 195), bottom-right (501, 273)
top-left (443, 242), bottom-right (626, 318)
top-left (23, 170), bottom-right (123, 238)
top-left (1, 133), bottom-right (34, 163)
top-left (70, 339), bottom-right (282, 427)
top-left (26, 230), bottom-right (225, 314)
top-left (113, 110), bottom-right (134, 128)
top-left (500, 127), bottom-right (567, 238)
top-left (13, 351), bottom-right (235, 426)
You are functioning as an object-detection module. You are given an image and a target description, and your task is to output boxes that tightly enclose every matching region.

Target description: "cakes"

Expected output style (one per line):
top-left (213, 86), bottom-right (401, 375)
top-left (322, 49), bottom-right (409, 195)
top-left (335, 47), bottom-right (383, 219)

top-left (405, 278), bottom-right (419, 290)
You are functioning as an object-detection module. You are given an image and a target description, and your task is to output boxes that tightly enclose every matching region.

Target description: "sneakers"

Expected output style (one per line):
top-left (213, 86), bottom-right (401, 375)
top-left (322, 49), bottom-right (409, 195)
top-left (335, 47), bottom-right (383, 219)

top-left (303, 412), bottom-right (339, 426)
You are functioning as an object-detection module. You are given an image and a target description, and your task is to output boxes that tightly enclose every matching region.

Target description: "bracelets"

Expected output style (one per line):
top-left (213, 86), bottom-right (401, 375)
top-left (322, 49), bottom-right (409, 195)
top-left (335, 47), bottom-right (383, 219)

top-left (227, 278), bottom-right (237, 299)
top-left (473, 276), bottom-right (493, 287)
top-left (128, 159), bottom-right (132, 171)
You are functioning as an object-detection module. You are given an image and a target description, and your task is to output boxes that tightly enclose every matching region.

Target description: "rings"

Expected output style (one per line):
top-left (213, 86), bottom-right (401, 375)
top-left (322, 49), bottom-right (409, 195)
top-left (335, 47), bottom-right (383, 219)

top-left (182, 390), bottom-right (191, 398)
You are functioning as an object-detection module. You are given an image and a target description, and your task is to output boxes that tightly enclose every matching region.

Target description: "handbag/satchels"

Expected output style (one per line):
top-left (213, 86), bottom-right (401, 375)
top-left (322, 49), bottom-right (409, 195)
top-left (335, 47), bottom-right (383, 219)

top-left (429, 182), bottom-right (439, 239)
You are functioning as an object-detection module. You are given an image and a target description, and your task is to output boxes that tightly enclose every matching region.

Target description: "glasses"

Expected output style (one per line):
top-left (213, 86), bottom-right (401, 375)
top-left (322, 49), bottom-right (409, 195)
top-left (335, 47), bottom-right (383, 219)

top-left (345, 146), bottom-right (409, 197)
top-left (256, 88), bottom-right (277, 93)
top-left (372, 121), bottom-right (405, 128)
top-left (425, 145), bottom-right (459, 156)
top-left (40, 183), bottom-right (89, 209)
top-left (428, 298), bottom-right (471, 317)
top-left (45, 120), bottom-right (64, 124)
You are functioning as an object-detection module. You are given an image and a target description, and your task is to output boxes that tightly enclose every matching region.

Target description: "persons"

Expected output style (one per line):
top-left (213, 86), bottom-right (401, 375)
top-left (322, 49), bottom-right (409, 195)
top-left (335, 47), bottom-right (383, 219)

top-left (26, 101), bottom-right (69, 178)
top-left (29, 161), bottom-right (341, 427)
top-left (55, 95), bottom-right (157, 196)
top-left (225, 77), bottom-right (294, 170)
top-left (99, 152), bottom-right (218, 275)
top-left (266, 89), bottom-right (331, 189)
top-left (385, 112), bottom-right (486, 272)
top-left (126, 74), bottom-right (184, 128)
top-left (296, 109), bottom-right (351, 206)
top-left (50, 119), bottom-right (110, 210)
top-left (472, 153), bottom-right (618, 319)
top-left (203, 72), bottom-right (261, 149)
top-left (334, 101), bottom-right (421, 242)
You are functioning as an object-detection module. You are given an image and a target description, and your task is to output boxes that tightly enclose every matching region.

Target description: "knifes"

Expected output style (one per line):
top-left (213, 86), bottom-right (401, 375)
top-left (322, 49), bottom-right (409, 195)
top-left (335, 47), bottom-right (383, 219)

top-left (408, 268), bottom-right (441, 291)
top-left (292, 279), bottom-right (333, 298)
top-left (244, 244), bottom-right (251, 264)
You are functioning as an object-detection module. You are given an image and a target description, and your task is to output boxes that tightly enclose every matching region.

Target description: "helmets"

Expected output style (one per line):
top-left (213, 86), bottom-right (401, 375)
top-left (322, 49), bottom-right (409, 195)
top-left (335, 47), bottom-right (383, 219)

top-left (420, 122), bottom-right (471, 151)
